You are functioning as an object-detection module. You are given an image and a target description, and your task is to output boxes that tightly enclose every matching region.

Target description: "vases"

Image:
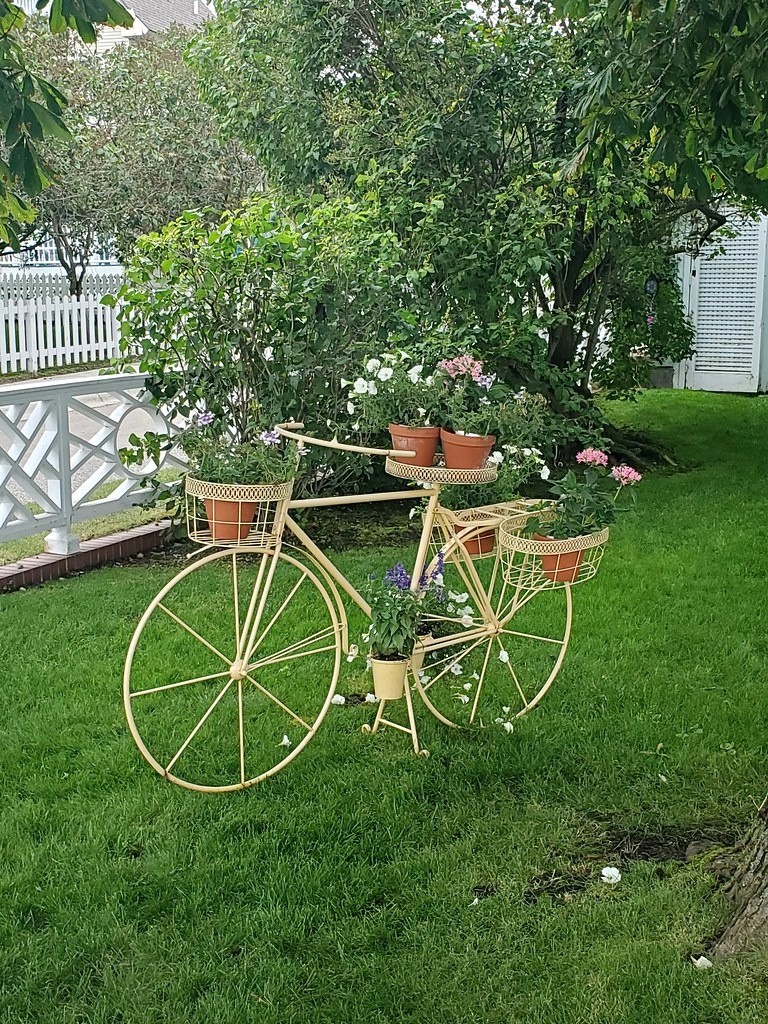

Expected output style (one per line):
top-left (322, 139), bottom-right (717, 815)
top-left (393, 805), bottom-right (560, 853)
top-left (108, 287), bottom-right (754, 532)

top-left (533, 535), bottom-right (585, 582)
top-left (454, 523), bottom-right (494, 554)
top-left (371, 660), bottom-right (407, 699)
top-left (388, 422), bottom-right (440, 467)
top-left (204, 499), bottom-right (258, 540)
top-left (440, 427), bottom-right (495, 468)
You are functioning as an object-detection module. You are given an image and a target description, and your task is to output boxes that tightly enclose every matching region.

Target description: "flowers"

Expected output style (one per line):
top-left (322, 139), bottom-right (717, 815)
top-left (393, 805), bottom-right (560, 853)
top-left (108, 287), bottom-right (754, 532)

top-left (363, 552), bottom-right (474, 655)
top-left (340, 349), bottom-right (642, 537)
top-left (175, 410), bottom-right (310, 486)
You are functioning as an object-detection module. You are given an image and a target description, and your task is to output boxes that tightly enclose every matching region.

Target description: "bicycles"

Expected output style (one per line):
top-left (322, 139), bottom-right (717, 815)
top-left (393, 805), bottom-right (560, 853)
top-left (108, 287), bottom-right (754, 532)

top-left (123, 421), bottom-right (611, 793)
